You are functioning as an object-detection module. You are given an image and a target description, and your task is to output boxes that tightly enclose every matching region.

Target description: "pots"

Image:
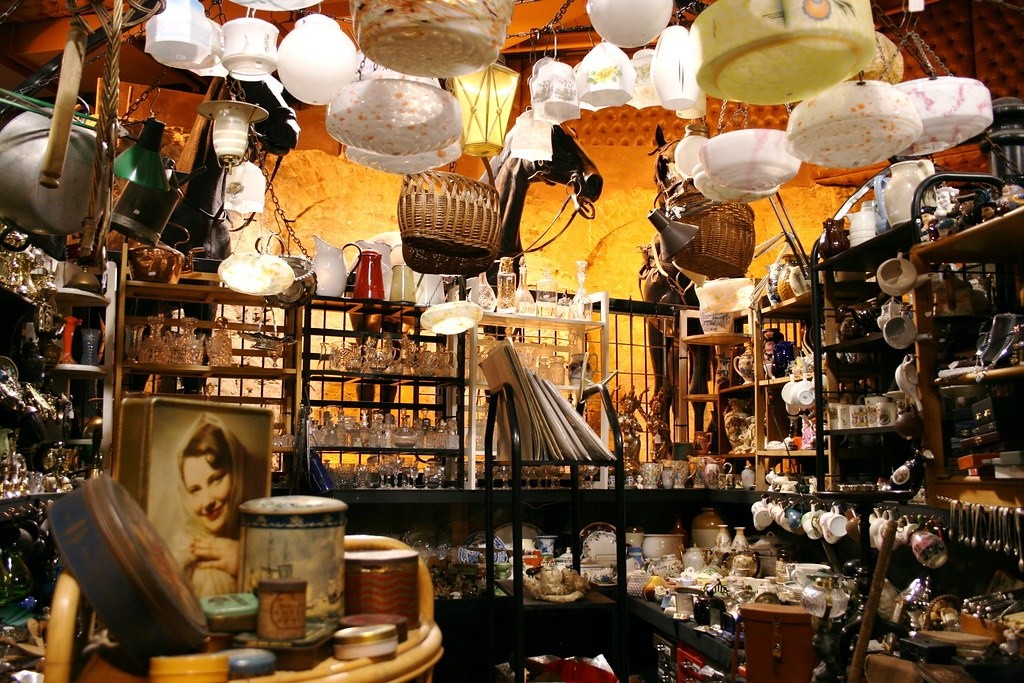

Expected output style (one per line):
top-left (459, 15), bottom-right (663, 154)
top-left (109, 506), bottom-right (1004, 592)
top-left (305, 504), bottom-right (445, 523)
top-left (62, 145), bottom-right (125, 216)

top-left (0, 95), bottom-right (108, 252)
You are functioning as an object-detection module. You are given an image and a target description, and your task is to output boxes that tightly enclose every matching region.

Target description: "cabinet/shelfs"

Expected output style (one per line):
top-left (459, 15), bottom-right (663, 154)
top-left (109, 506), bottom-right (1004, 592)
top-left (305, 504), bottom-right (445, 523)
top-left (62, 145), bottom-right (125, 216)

top-left (0, 206), bottom-right (1024, 683)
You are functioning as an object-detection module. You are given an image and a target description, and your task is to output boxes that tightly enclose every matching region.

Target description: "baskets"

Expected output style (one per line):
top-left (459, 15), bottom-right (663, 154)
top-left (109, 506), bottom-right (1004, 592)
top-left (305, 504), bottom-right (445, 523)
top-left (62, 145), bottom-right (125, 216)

top-left (397, 156), bottom-right (501, 275)
top-left (665, 178), bottom-right (755, 278)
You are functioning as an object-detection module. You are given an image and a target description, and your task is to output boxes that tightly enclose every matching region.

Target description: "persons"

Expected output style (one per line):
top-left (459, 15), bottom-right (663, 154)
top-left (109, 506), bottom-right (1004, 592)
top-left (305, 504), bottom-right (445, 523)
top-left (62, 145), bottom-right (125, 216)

top-left (164, 412), bottom-right (256, 599)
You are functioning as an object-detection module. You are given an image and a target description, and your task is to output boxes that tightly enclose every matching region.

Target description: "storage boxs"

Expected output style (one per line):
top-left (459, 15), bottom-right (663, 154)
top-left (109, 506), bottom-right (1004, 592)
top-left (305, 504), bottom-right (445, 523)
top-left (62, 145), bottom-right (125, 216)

top-left (970, 395), bottom-right (1016, 426)
top-left (839, 452), bottom-right (877, 483)
top-left (952, 408), bottom-right (973, 429)
top-left (857, 362), bottom-right (892, 395)
top-left (956, 452), bottom-right (998, 470)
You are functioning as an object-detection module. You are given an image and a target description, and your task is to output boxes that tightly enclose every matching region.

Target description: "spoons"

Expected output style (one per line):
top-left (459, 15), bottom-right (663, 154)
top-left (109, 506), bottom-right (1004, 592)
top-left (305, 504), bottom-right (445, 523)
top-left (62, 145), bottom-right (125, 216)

top-left (948, 497), bottom-right (1024, 572)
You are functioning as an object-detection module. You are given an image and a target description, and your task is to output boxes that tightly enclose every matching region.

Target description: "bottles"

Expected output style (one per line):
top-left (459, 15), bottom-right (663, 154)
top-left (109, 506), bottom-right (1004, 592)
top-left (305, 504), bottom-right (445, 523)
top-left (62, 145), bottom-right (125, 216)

top-left (259, 580), bottom-right (307, 639)
top-left (691, 507), bottom-right (747, 550)
top-left (477, 336), bottom-right (565, 386)
top-left (140, 314), bottom-right (232, 367)
top-left (311, 407), bottom-right (459, 449)
top-left (468, 257), bottom-right (593, 320)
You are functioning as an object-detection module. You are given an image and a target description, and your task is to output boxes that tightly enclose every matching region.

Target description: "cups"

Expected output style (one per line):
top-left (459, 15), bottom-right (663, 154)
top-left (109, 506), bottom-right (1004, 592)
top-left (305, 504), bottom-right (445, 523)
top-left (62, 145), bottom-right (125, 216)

top-left (781, 373), bottom-right (815, 405)
top-left (693, 431), bottom-right (712, 454)
top-left (747, 498), bottom-right (947, 568)
top-left (829, 397), bottom-right (895, 430)
top-left (125, 324), bottom-right (145, 363)
top-left (80, 329), bottom-right (101, 366)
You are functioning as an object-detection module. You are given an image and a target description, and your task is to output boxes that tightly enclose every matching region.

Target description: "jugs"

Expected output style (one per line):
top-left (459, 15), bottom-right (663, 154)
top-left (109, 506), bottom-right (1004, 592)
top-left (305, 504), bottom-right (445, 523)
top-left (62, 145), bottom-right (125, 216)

top-left (309, 235), bottom-right (444, 306)
top-left (819, 160), bottom-right (935, 259)
top-left (640, 456), bottom-right (729, 490)
top-left (733, 346), bottom-right (754, 385)
top-left (569, 352), bottom-right (598, 385)
top-left (125, 221), bottom-right (203, 284)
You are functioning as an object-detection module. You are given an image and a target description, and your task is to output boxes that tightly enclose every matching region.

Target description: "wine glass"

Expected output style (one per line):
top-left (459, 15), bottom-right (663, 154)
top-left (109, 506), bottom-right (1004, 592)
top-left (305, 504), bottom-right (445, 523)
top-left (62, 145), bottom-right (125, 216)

top-left (323, 456), bottom-right (600, 490)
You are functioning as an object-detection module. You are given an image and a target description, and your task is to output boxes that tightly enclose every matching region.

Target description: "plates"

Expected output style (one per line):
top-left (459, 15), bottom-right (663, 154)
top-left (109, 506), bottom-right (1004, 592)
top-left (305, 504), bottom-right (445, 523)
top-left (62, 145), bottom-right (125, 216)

top-left (401, 522), bottom-right (617, 562)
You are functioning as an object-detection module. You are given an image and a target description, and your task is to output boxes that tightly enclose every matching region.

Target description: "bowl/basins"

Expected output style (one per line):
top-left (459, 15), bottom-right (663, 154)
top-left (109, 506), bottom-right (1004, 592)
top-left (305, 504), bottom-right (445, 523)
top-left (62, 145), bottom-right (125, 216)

top-left (642, 534), bottom-right (686, 558)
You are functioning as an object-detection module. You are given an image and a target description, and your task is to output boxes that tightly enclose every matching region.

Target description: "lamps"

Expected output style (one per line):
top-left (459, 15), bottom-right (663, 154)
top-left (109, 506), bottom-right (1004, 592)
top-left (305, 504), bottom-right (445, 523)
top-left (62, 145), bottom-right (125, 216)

top-left (647, 209), bottom-right (700, 256)
top-left (140, 1), bottom-right (993, 336)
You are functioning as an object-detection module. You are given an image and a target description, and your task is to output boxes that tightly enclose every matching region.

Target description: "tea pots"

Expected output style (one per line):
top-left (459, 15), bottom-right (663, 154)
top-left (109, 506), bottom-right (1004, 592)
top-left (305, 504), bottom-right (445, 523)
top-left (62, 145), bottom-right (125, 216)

top-left (677, 542), bottom-right (762, 577)
top-left (876, 252), bottom-right (939, 411)
top-left (318, 340), bottom-right (457, 377)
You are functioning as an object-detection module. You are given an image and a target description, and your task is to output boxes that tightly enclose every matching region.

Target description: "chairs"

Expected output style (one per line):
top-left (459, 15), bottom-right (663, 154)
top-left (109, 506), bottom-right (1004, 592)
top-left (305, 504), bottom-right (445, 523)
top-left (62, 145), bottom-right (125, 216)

top-left (40, 567), bottom-right (94, 683)
top-left (340, 533), bottom-right (434, 624)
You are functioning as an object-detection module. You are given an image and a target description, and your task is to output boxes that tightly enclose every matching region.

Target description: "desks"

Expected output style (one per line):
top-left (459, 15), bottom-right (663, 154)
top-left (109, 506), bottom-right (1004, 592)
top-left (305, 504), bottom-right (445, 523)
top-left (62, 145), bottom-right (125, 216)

top-left (74, 621), bottom-right (443, 683)
